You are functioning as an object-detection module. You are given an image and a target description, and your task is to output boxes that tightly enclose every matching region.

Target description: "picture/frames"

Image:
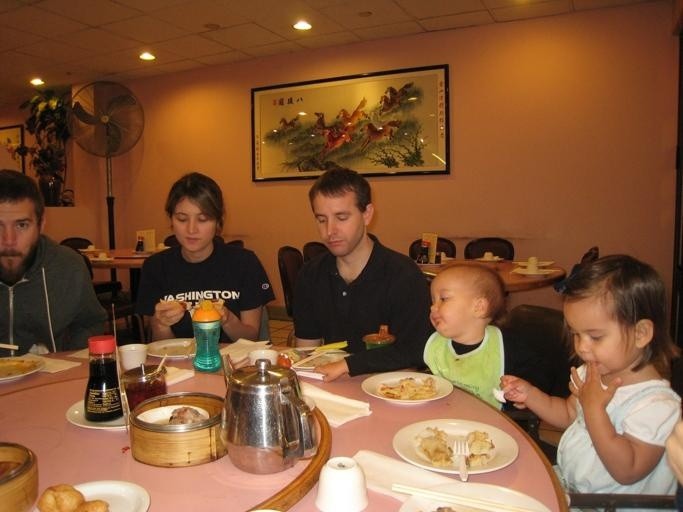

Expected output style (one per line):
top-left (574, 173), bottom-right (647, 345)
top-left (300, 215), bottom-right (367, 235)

top-left (249, 64), bottom-right (451, 182)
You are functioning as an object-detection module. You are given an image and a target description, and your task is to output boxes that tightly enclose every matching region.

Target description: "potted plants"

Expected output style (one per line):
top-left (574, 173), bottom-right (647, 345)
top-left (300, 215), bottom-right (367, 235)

top-left (7, 87), bottom-right (73, 207)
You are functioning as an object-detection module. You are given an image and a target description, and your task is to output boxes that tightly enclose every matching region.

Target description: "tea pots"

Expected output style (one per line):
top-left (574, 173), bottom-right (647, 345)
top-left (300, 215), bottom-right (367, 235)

top-left (220, 353), bottom-right (318, 475)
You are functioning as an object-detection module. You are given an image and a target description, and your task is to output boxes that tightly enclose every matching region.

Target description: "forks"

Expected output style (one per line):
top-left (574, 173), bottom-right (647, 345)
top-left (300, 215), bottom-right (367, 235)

top-left (453, 440), bottom-right (471, 482)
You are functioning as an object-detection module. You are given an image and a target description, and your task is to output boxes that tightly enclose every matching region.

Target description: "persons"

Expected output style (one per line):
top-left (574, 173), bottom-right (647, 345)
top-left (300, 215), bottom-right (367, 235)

top-left (316, 263), bottom-right (521, 412)
top-left (136, 171), bottom-right (276, 343)
top-left (0, 169), bottom-right (110, 351)
top-left (290, 166), bottom-right (427, 349)
top-left (500, 247), bottom-right (681, 495)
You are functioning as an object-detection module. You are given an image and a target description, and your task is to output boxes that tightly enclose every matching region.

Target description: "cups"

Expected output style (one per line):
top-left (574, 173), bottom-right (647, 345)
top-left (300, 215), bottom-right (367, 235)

top-left (119, 343), bottom-right (148, 371)
top-left (158, 243), bottom-right (165, 249)
top-left (99, 253), bottom-right (107, 259)
top-left (441, 252), bottom-right (446, 259)
top-left (191, 299), bottom-right (220, 375)
top-left (314, 456), bottom-right (368, 512)
top-left (88, 245), bottom-right (96, 251)
top-left (484, 251), bottom-right (494, 260)
top-left (250, 348), bottom-right (277, 368)
top-left (363, 333), bottom-right (396, 349)
top-left (527, 257), bottom-right (539, 272)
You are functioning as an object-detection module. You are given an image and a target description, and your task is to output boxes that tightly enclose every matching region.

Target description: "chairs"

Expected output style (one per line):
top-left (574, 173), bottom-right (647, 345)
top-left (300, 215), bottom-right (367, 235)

top-left (277, 247), bottom-right (302, 316)
top-left (500, 305), bottom-right (569, 398)
top-left (303, 241), bottom-right (329, 261)
top-left (409, 238), bottom-right (456, 260)
top-left (59, 238), bottom-right (94, 281)
top-left (464, 237), bottom-right (514, 259)
top-left (503, 357), bottom-right (682, 512)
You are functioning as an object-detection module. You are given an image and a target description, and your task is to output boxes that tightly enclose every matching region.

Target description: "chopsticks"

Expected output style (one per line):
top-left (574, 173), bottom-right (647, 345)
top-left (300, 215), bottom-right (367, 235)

top-left (291, 342), bottom-right (334, 367)
top-left (391, 484), bottom-right (534, 512)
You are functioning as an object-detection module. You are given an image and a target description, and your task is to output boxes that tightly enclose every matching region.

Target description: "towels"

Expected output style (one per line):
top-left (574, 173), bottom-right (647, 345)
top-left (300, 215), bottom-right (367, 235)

top-left (163, 365), bottom-right (196, 387)
top-left (219, 337), bottom-right (273, 364)
top-left (21, 353), bottom-right (81, 374)
top-left (300, 380), bottom-right (372, 430)
top-left (353, 449), bottom-right (463, 502)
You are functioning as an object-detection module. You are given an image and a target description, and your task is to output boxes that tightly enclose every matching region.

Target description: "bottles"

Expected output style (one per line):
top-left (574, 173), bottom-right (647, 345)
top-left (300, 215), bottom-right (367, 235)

top-left (136, 235), bottom-right (144, 252)
top-left (192, 302), bottom-right (224, 371)
top-left (419, 241), bottom-right (430, 263)
top-left (84, 335), bottom-right (122, 420)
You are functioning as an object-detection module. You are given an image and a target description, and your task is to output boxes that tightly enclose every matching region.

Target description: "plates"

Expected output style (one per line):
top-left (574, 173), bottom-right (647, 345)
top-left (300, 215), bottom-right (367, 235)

top-left (0, 357), bottom-right (45, 382)
top-left (392, 418), bottom-right (520, 475)
top-left (279, 346), bottom-right (346, 371)
top-left (518, 262), bottom-right (550, 266)
top-left (79, 248), bottom-right (100, 252)
top-left (362, 372), bottom-right (454, 405)
top-left (137, 402), bottom-right (208, 425)
top-left (415, 260), bottom-right (442, 267)
top-left (32, 480), bottom-right (150, 512)
top-left (441, 257), bottom-right (454, 260)
top-left (477, 257), bottom-right (504, 261)
top-left (513, 267), bottom-right (551, 277)
top-left (146, 338), bottom-right (196, 360)
top-left (397, 482), bottom-right (552, 512)
top-left (66, 399), bottom-right (132, 431)
top-left (90, 256), bottom-right (114, 262)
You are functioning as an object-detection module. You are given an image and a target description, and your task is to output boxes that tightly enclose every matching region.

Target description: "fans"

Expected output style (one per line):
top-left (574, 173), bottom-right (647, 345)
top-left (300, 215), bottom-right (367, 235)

top-left (68, 81), bottom-right (146, 306)
